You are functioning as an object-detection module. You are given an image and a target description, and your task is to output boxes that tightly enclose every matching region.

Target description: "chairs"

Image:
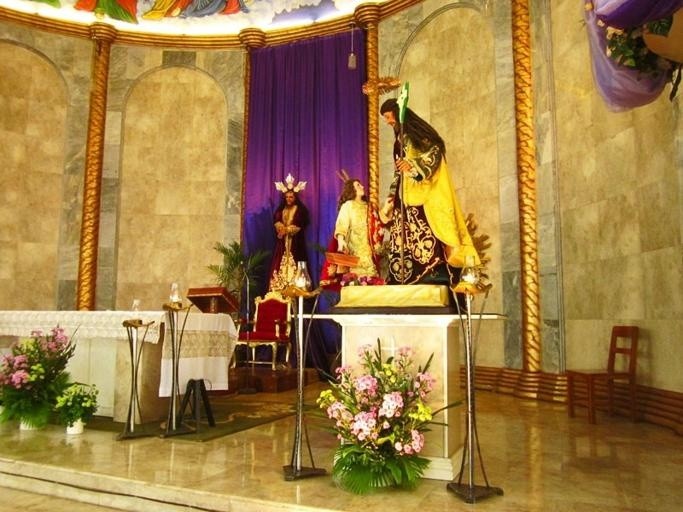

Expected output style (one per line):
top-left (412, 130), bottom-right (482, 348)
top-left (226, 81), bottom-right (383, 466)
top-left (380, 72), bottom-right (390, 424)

top-left (230, 290), bottom-right (293, 370)
top-left (566, 326), bottom-right (640, 422)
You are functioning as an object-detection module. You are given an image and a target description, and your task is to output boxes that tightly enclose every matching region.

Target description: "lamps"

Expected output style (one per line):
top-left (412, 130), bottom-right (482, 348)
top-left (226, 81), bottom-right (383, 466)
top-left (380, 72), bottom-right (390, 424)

top-left (118, 299), bottom-right (155, 439)
top-left (282, 258), bottom-right (326, 481)
top-left (157, 281), bottom-right (196, 437)
top-left (448, 254), bottom-right (504, 499)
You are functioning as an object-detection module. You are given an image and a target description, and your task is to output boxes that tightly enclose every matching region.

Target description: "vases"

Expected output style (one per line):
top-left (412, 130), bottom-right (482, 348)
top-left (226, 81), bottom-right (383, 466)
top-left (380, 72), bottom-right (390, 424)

top-left (65, 417), bottom-right (88, 434)
top-left (17, 418), bottom-right (49, 431)
top-left (369, 470), bottom-right (395, 488)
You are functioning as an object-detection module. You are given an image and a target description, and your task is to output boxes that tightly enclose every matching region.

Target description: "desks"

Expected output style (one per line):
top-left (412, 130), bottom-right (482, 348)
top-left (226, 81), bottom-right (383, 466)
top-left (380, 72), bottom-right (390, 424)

top-left (0, 310), bottom-right (239, 424)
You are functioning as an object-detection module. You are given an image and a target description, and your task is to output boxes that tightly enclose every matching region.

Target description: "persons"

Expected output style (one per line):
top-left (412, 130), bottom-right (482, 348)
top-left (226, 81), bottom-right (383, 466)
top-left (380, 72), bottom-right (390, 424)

top-left (266, 174), bottom-right (312, 298)
top-left (320, 179), bottom-right (384, 287)
top-left (379, 99), bottom-right (480, 284)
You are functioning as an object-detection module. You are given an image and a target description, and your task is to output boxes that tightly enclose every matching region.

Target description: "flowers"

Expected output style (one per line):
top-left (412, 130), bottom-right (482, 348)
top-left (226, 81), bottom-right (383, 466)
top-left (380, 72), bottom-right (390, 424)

top-left (311, 336), bottom-right (465, 498)
top-left (0, 317), bottom-right (81, 432)
top-left (52, 381), bottom-right (101, 426)
